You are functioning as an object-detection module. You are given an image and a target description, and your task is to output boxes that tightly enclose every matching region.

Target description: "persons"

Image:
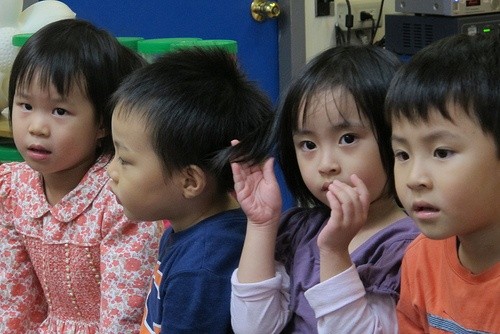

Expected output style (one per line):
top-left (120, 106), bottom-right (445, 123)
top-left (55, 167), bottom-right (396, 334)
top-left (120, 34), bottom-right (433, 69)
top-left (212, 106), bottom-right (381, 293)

top-left (230, 43), bottom-right (421, 334)
top-left (0, 18), bottom-right (166, 334)
top-left (106, 44), bottom-right (274, 334)
top-left (383, 31), bottom-right (500, 334)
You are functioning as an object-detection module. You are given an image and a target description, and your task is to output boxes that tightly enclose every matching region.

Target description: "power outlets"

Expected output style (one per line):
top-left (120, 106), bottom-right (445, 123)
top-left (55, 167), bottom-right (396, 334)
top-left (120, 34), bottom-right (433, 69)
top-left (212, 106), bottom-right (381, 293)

top-left (359, 9), bottom-right (376, 23)
